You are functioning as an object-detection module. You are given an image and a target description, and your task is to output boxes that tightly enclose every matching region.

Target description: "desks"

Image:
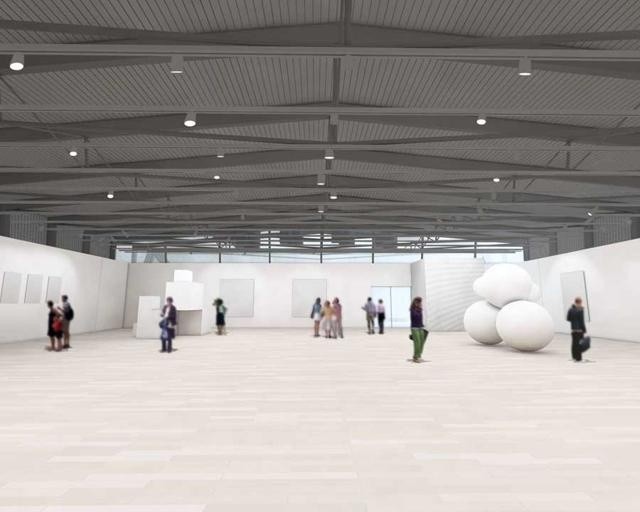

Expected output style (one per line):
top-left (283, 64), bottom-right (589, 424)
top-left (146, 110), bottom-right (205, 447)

top-left (169, 308), bottom-right (209, 335)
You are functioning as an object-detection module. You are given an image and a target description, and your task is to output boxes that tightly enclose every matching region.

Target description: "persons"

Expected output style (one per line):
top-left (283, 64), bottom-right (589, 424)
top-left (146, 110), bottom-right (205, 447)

top-left (160, 297), bottom-right (178, 328)
top-left (310, 297), bottom-right (323, 337)
top-left (212, 298), bottom-right (227, 335)
top-left (159, 316), bottom-right (176, 353)
top-left (320, 301), bottom-right (336, 337)
top-left (44, 301), bottom-right (62, 352)
top-left (376, 299), bottom-right (386, 334)
top-left (57, 294), bottom-right (75, 350)
top-left (567, 295), bottom-right (588, 364)
top-left (362, 296), bottom-right (376, 334)
top-left (331, 297), bottom-right (344, 339)
top-left (409, 296), bottom-right (425, 363)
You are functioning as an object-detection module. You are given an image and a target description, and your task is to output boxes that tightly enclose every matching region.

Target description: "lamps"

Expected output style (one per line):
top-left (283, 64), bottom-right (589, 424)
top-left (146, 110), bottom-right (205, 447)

top-left (183, 112), bottom-right (196, 127)
top-left (10, 53), bottom-right (25, 70)
top-left (517, 55), bottom-right (532, 76)
top-left (329, 191), bottom-right (338, 200)
top-left (69, 144), bottom-right (77, 157)
top-left (476, 116), bottom-right (487, 125)
top-left (324, 149), bottom-right (335, 160)
top-left (170, 55), bottom-right (183, 74)
top-left (316, 174), bottom-right (325, 185)
top-left (106, 188), bottom-right (115, 199)
top-left (215, 149), bottom-right (224, 158)
